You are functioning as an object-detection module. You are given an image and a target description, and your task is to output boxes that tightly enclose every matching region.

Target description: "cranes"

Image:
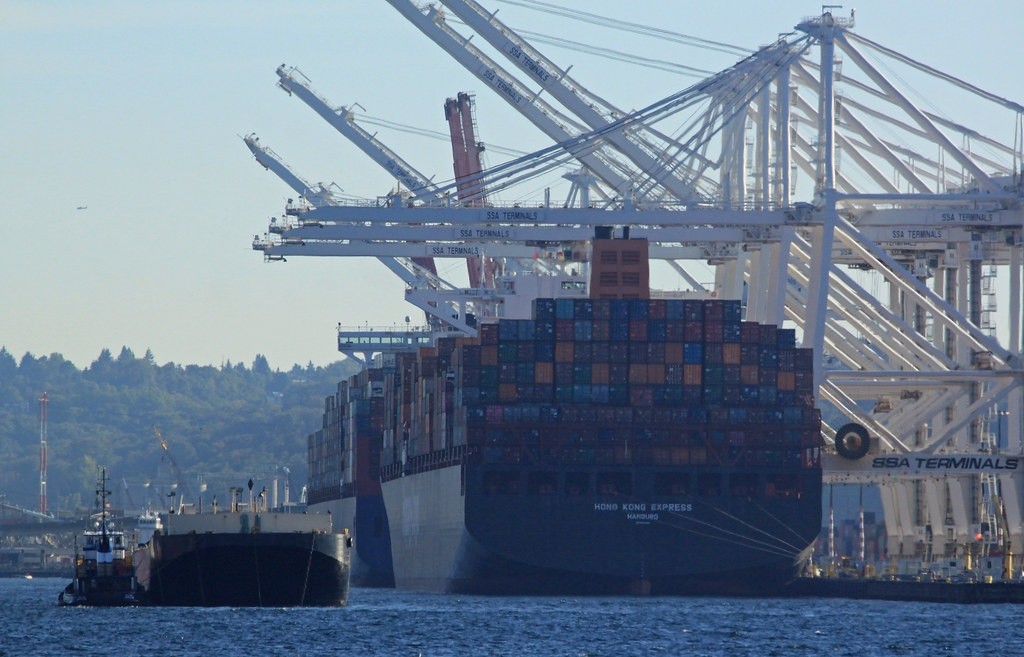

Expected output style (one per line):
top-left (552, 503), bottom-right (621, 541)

top-left (154, 424), bottom-right (196, 505)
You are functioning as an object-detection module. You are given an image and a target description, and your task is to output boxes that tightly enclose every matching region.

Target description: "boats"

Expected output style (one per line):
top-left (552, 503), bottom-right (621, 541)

top-left (299, 232), bottom-right (824, 589)
top-left (58, 468), bottom-right (142, 608)
top-left (126, 465), bottom-right (353, 603)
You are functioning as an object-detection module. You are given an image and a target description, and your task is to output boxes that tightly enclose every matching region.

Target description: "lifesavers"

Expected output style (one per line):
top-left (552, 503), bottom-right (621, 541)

top-left (835, 423), bottom-right (871, 460)
